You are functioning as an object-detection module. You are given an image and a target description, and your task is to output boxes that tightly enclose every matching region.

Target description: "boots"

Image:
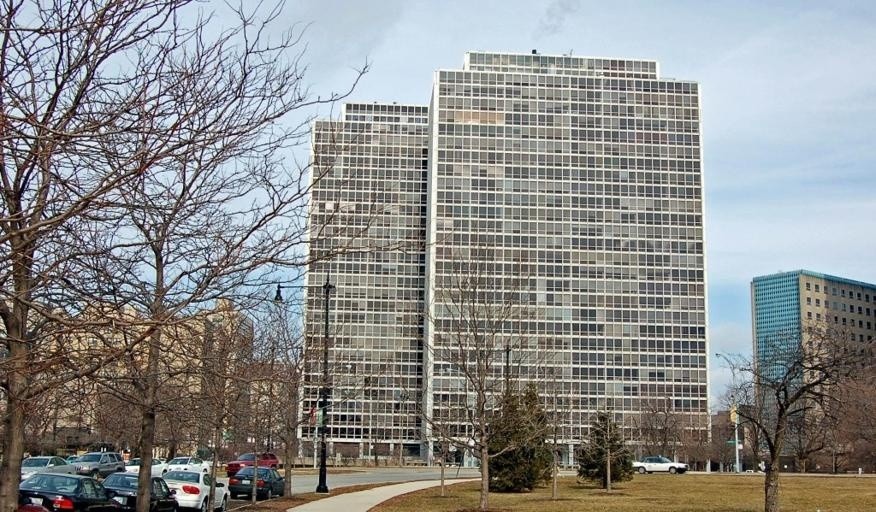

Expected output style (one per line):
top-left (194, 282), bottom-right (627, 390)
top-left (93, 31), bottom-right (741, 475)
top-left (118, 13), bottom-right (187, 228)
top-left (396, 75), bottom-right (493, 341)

top-left (226, 451), bottom-right (280, 477)
top-left (69, 450), bottom-right (126, 479)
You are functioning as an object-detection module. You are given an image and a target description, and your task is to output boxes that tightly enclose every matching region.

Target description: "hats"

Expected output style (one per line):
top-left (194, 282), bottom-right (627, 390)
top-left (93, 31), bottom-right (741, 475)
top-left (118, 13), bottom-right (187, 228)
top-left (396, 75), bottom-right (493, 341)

top-left (65, 455), bottom-right (79, 463)
top-left (166, 456), bottom-right (209, 476)
top-left (630, 455), bottom-right (690, 475)
top-left (14, 471), bottom-right (121, 510)
top-left (19, 456), bottom-right (75, 481)
top-left (229, 465), bottom-right (286, 500)
top-left (100, 472), bottom-right (178, 511)
top-left (123, 457), bottom-right (169, 477)
top-left (157, 469), bottom-right (228, 512)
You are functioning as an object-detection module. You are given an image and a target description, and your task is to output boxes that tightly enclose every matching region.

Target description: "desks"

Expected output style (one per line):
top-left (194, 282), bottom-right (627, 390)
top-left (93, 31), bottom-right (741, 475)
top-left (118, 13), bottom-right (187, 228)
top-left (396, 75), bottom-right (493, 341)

top-left (267, 343), bottom-right (305, 370)
top-left (271, 269), bottom-right (333, 495)
top-left (482, 339), bottom-right (512, 406)
top-left (713, 352), bottom-right (739, 473)
